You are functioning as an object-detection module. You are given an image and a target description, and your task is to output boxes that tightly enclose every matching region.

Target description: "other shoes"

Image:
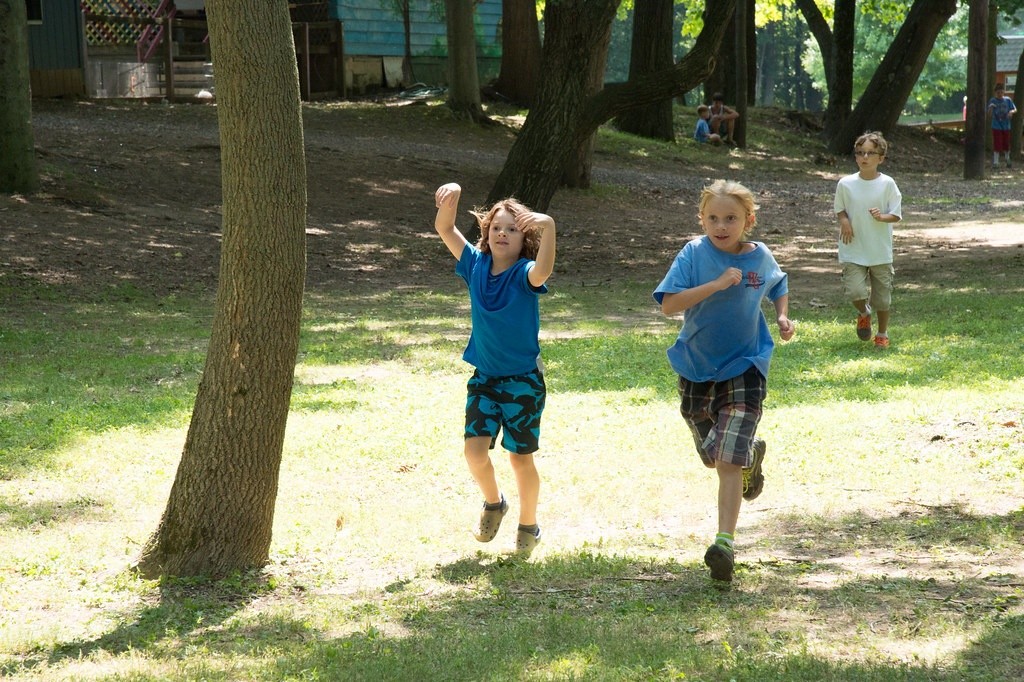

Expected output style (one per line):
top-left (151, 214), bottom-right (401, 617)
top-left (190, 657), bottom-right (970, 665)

top-left (993, 163), bottom-right (999, 168)
top-left (1006, 164), bottom-right (1012, 170)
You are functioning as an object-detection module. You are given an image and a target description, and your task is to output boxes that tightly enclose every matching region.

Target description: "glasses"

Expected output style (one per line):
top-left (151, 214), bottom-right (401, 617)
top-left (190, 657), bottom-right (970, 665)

top-left (855, 150), bottom-right (880, 155)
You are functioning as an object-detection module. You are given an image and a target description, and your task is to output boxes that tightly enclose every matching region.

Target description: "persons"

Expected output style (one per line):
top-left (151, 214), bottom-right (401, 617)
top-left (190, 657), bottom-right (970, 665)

top-left (708, 91), bottom-right (742, 147)
top-left (692, 102), bottom-right (723, 147)
top-left (645, 180), bottom-right (799, 583)
top-left (829, 132), bottom-right (905, 349)
top-left (431, 180), bottom-right (560, 555)
top-left (987, 80), bottom-right (1017, 169)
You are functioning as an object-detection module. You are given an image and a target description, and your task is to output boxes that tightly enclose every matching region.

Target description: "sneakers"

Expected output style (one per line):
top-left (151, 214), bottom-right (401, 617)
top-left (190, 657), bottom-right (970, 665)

top-left (874, 335), bottom-right (890, 348)
top-left (857, 304), bottom-right (872, 341)
top-left (705, 545), bottom-right (735, 581)
top-left (742, 437), bottom-right (766, 501)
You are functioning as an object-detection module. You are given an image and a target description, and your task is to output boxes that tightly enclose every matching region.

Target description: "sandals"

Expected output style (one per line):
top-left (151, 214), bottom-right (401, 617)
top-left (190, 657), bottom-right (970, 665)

top-left (473, 492), bottom-right (509, 542)
top-left (515, 528), bottom-right (542, 560)
